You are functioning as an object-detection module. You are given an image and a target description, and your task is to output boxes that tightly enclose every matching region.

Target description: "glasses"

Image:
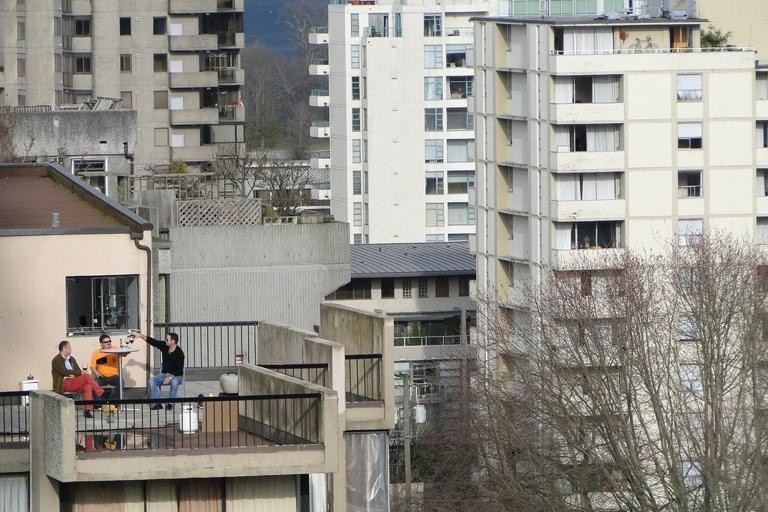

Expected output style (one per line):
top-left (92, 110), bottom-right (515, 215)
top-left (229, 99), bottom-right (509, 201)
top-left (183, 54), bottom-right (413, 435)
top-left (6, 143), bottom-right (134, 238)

top-left (102, 340), bottom-right (111, 343)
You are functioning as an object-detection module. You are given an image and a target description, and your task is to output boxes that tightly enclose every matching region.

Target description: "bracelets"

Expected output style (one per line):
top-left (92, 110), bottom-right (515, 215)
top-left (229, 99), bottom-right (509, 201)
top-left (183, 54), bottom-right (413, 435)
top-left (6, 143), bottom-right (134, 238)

top-left (169, 375), bottom-right (172, 379)
top-left (94, 372), bottom-right (99, 375)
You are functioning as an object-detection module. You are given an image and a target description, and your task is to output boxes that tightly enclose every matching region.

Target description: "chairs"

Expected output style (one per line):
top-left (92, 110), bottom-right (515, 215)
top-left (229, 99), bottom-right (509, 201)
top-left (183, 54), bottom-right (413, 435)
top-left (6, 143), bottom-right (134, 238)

top-left (157, 359), bottom-right (187, 408)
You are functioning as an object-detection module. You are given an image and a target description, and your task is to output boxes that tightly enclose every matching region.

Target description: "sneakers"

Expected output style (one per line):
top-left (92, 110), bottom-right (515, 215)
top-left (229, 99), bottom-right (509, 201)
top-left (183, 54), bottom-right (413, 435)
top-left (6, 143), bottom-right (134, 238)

top-left (101, 388), bottom-right (112, 399)
top-left (152, 403), bottom-right (163, 410)
top-left (84, 410), bottom-right (93, 418)
top-left (166, 404), bottom-right (172, 410)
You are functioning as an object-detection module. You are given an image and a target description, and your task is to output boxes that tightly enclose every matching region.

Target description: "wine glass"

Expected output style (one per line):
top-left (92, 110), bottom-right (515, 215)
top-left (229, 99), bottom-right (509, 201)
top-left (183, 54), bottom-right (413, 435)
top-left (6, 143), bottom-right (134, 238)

top-left (83, 363), bottom-right (88, 371)
top-left (122, 335), bottom-right (134, 350)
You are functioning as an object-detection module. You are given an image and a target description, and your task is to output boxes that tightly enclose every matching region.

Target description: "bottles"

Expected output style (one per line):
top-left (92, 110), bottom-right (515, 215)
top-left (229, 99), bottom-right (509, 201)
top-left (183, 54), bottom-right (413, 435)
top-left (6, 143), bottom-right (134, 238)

top-left (127, 329), bottom-right (140, 334)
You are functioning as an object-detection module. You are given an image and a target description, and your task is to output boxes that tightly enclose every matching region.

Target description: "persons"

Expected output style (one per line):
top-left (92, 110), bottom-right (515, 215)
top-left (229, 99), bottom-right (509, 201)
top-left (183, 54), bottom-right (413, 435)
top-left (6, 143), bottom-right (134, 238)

top-left (90, 335), bottom-right (130, 407)
top-left (51, 341), bottom-right (113, 418)
top-left (127, 331), bottom-right (183, 410)
